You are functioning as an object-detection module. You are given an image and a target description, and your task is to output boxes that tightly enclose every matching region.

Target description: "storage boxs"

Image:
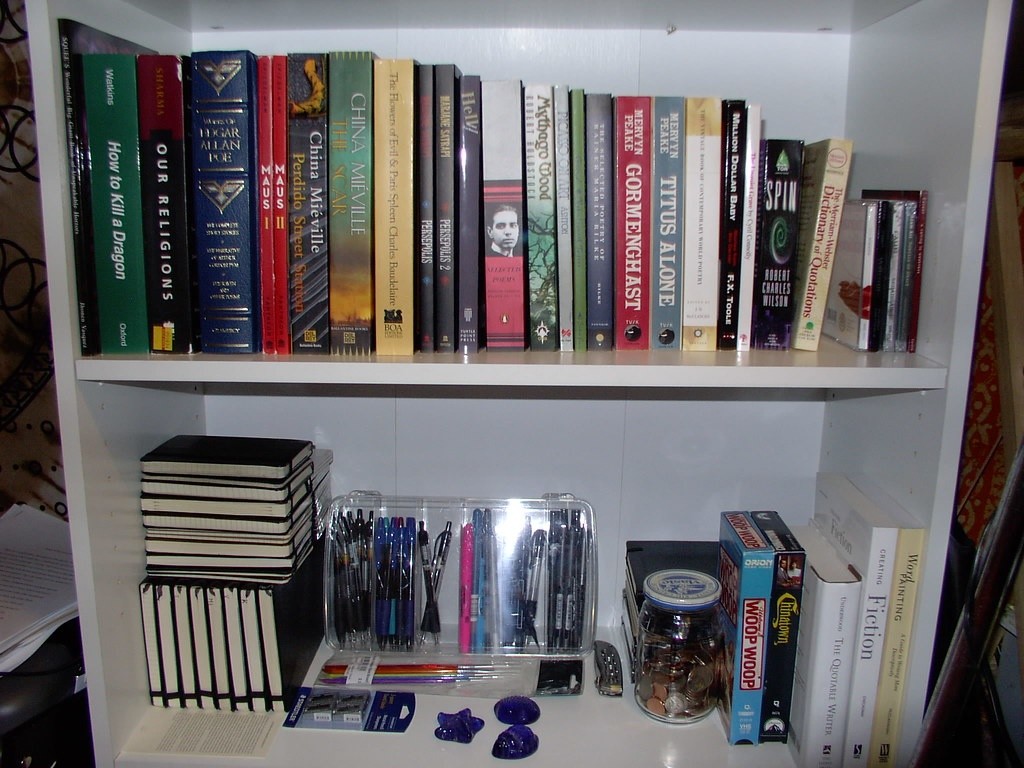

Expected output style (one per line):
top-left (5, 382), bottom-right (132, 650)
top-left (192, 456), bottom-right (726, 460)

top-left (719, 510), bottom-right (808, 747)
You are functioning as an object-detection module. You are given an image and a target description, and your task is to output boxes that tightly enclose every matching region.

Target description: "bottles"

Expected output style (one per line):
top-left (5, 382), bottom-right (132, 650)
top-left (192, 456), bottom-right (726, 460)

top-left (635, 569), bottom-right (724, 723)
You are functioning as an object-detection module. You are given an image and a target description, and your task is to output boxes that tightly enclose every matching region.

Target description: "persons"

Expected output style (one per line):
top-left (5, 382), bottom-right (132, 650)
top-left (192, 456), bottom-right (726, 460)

top-left (487, 204), bottom-right (519, 257)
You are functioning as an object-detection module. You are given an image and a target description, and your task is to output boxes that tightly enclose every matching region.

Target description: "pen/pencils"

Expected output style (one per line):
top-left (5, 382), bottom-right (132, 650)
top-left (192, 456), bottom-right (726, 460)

top-left (324, 491), bottom-right (593, 656)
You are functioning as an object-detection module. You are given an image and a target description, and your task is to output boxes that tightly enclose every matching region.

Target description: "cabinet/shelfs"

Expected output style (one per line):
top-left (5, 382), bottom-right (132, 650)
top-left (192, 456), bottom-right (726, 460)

top-left (23, 0), bottom-right (1013, 768)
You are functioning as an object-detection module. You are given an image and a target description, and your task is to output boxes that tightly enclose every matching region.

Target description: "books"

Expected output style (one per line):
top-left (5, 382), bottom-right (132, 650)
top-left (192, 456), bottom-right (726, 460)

top-left (58, 19), bottom-right (926, 768)
